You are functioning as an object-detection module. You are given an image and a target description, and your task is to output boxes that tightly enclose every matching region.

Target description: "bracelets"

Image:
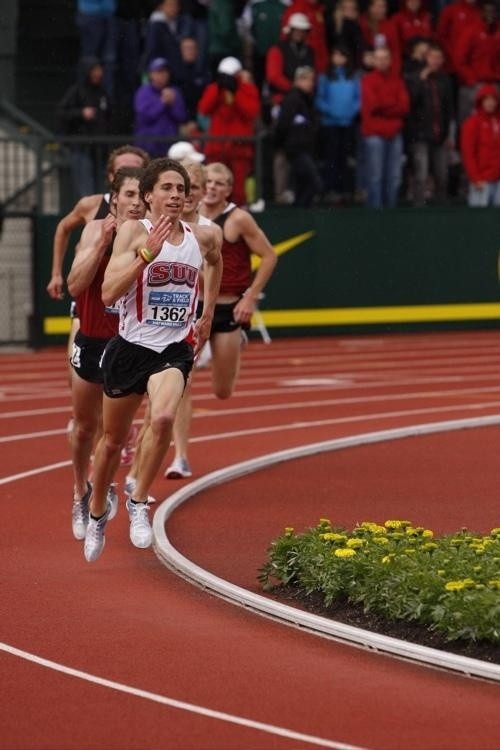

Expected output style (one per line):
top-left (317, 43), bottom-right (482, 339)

top-left (140, 253), bottom-right (149, 264)
top-left (143, 247), bottom-right (154, 261)
top-left (141, 248), bottom-right (152, 263)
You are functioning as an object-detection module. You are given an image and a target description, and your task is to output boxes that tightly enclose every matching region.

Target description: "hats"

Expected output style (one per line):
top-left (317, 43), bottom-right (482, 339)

top-left (168, 142), bottom-right (206, 164)
top-left (217, 57), bottom-right (242, 74)
top-left (150, 58), bottom-right (171, 73)
top-left (285, 13), bottom-right (313, 30)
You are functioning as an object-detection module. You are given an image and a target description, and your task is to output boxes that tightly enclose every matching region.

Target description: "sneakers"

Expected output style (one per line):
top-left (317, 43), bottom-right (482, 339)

top-left (120, 429), bottom-right (138, 466)
top-left (164, 458), bottom-right (193, 477)
top-left (67, 473), bottom-right (155, 561)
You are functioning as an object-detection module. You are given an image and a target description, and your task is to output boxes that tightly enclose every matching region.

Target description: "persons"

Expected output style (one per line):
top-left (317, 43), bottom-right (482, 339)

top-left (162, 161), bottom-right (280, 482)
top-left (49, 0), bottom-right (499, 213)
top-left (119, 155), bottom-right (219, 508)
top-left (43, 141), bottom-right (150, 466)
top-left (64, 167), bottom-right (154, 539)
top-left (80, 156), bottom-right (226, 566)
